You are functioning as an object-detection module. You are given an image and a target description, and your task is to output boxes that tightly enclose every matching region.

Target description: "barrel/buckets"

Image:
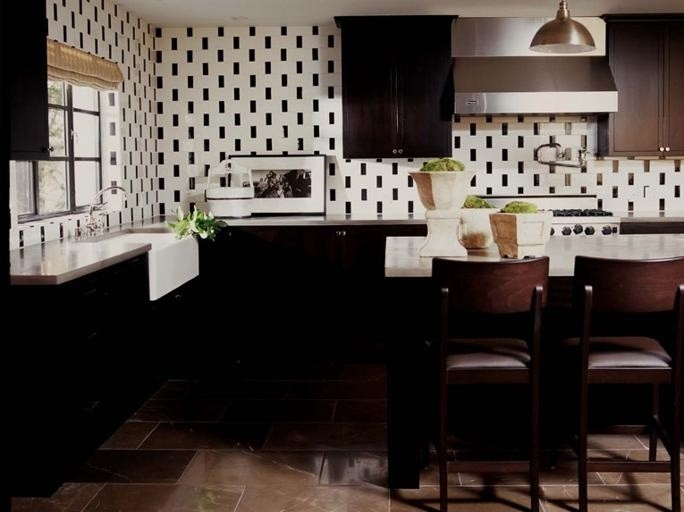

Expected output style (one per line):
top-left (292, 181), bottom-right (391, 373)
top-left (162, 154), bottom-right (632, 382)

top-left (207, 160), bottom-right (256, 219)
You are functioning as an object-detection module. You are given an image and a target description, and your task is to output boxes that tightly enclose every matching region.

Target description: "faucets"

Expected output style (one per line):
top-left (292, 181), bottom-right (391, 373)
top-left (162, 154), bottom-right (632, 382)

top-left (86, 186), bottom-right (128, 236)
top-left (533, 141), bottom-right (585, 169)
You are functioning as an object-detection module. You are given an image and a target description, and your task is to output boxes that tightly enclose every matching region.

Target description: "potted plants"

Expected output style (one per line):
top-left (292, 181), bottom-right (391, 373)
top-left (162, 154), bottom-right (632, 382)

top-left (461, 196), bottom-right (499, 248)
top-left (488, 201), bottom-right (553, 258)
top-left (408, 159), bottom-right (476, 258)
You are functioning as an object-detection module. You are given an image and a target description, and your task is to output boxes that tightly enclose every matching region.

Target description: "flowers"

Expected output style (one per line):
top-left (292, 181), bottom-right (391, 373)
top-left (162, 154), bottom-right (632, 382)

top-left (165, 204), bottom-right (227, 244)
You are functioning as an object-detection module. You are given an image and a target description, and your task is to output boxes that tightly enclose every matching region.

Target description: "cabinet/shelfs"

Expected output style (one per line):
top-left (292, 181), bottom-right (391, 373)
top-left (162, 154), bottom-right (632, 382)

top-left (8, 250), bottom-right (151, 496)
top-left (197, 227), bottom-right (275, 425)
top-left (0, 0), bottom-right (52, 162)
top-left (595, 14), bottom-right (684, 160)
top-left (275, 225), bottom-right (428, 426)
top-left (149, 276), bottom-right (197, 392)
top-left (334, 14), bottom-right (458, 158)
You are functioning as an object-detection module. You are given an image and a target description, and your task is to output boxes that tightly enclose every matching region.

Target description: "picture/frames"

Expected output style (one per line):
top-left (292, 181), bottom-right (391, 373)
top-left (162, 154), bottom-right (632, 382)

top-left (227, 154), bottom-right (327, 217)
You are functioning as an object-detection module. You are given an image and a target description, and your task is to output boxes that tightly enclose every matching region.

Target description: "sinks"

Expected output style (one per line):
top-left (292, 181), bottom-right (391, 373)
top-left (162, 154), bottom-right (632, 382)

top-left (74, 227), bottom-right (202, 304)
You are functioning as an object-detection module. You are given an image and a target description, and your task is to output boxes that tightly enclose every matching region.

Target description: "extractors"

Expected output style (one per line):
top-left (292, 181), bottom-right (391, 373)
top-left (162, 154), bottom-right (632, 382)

top-left (449, 15), bottom-right (618, 115)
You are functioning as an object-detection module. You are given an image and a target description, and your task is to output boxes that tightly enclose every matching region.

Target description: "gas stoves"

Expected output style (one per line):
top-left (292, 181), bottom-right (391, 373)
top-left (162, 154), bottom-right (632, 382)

top-left (538, 208), bottom-right (620, 235)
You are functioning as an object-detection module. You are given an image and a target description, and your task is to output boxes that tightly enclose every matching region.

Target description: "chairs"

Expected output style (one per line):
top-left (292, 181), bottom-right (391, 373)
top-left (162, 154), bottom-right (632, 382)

top-left (434, 256), bottom-right (548, 512)
top-left (558, 255), bottom-right (683, 512)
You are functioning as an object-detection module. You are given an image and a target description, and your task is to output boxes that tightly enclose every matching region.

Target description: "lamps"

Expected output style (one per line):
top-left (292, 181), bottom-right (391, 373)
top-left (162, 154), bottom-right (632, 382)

top-left (530, 0), bottom-right (595, 53)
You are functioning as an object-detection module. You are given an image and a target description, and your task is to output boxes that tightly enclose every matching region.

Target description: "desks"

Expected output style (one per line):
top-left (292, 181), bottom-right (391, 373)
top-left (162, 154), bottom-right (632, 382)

top-left (384, 231), bottom-right (683, 491)
